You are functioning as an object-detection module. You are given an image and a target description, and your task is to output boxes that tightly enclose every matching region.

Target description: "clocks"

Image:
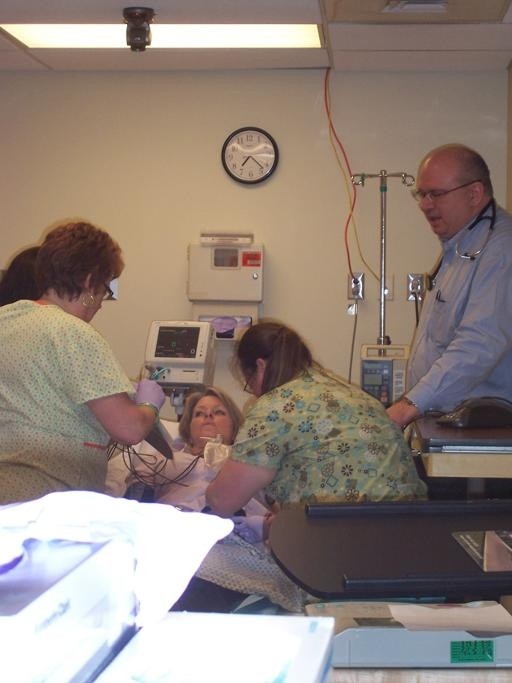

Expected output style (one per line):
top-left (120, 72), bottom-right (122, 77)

top-left (221, 126), bottom-right (279, 184)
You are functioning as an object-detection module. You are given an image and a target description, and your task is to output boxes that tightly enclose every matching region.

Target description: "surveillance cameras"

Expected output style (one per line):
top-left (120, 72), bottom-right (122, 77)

top-left (120, 8), bottom-right (156, 54)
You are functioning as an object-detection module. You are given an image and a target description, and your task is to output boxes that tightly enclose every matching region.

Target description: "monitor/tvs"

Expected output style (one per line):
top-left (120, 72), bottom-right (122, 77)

top-left (149, 322), bottom-right (202, 360)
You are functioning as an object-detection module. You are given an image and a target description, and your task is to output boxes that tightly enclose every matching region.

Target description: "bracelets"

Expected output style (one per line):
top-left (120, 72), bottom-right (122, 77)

top-left (402, 397), bottom-right (420, 409)
top-left (139, 402), bottom-right (160, 426)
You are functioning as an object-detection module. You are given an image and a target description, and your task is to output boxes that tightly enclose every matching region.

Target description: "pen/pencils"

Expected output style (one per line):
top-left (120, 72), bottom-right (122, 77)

top-left (85, 624), bottom-right (135, 683)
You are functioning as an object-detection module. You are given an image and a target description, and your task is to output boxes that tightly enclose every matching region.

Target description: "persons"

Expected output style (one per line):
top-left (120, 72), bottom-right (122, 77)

top-left (122, 383), bottom-right (243, 509)
top-left (383, 141), bottom-right (512, 428)
top-left (0, 217), bottom-right (167, 509)
top-left (202, 317), bottom-right (429, 547)
top-left (0, 244), bottom-right (46, 309)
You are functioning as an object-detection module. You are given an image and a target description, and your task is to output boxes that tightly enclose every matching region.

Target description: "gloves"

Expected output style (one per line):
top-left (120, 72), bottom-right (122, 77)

top-left (231, 515), bottom-right (264, 543)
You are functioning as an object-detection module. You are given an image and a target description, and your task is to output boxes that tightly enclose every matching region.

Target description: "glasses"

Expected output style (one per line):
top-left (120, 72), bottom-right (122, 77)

top-left (410, 179), bottom-right (482, 201)
top-left (242, 376), bottom-right (254, 394)
top-left (102, 280), bottom-right (114, 300)
